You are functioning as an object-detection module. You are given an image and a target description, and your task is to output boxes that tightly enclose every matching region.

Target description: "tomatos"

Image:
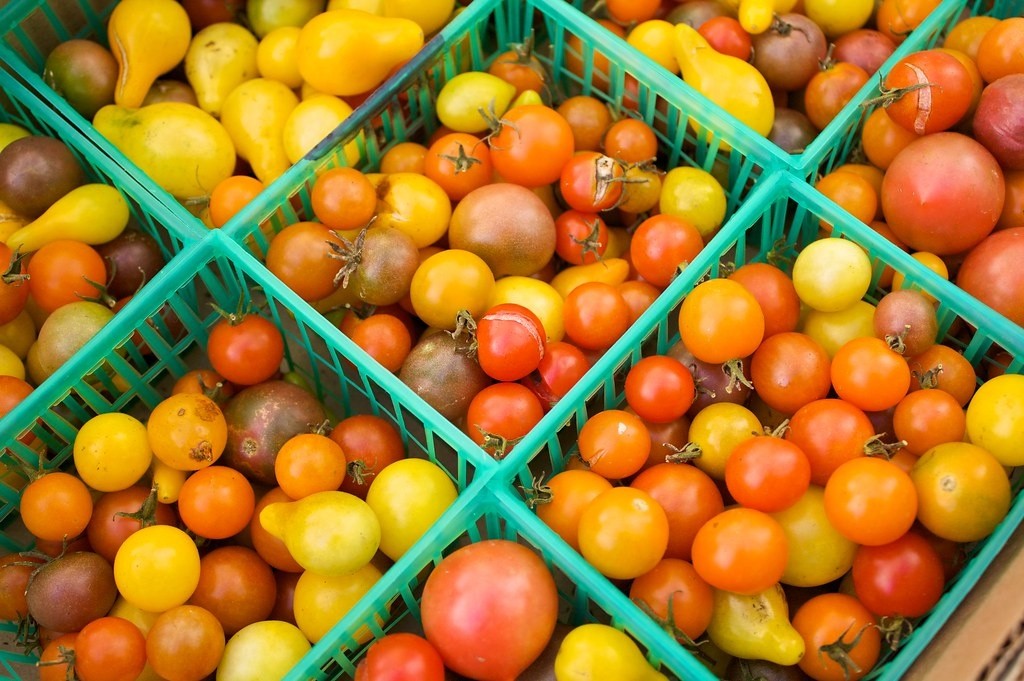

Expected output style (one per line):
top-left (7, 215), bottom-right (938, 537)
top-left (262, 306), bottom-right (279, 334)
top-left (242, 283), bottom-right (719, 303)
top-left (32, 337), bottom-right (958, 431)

top-left (0, 0), bottom-right (1024, 681)
